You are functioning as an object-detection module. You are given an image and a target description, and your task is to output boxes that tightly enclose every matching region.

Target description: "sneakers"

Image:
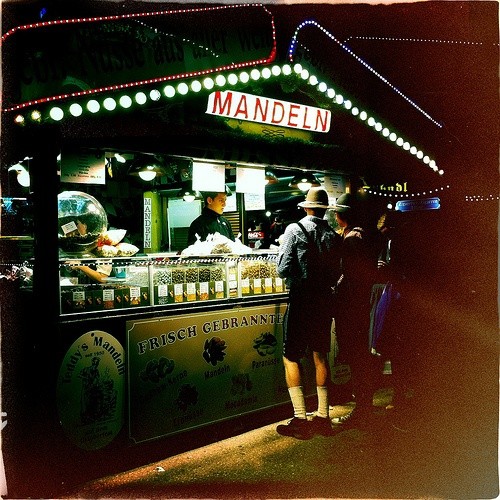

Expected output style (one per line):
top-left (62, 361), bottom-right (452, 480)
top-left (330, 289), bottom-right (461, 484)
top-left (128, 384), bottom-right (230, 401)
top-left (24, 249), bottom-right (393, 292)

top-left (310, 416), bottom-right (332, 437)
top-left (277, 417), bottom-right (315, 440)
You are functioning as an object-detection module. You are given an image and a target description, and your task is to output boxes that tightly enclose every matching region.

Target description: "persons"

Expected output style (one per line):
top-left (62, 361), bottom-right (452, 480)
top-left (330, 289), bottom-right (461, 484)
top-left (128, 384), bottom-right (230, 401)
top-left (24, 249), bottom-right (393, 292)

top-left (64, 213), bottom-right (116, 284)
top-left (333, 194), bottom-right (437, 432)
top-left (187, 192), bottom-right (235, 246)
top-left (276, 187), bottom-right (341, 439)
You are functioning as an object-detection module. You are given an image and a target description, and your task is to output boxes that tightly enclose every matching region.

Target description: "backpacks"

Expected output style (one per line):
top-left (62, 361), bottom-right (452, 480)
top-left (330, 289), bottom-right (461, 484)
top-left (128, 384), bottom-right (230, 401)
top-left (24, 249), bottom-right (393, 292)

top-left (296, 221), bottom-right (342, 311)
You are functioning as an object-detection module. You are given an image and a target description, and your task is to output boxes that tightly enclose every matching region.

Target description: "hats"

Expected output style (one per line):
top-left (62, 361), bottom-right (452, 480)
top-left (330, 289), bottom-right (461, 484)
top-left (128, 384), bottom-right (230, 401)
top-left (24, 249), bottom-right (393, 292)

top-left (329, 192), bottom-right (366, 214)
top-left (298, 188), bottom-right (336, 209)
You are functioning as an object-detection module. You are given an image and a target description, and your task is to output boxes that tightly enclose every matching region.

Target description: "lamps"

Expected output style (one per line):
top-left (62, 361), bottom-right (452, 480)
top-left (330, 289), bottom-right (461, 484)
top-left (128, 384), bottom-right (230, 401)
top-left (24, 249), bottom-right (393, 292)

top-left (7, 160), bottom-right (30, 188)
top-left (127, 154), bottom-right (162, 181)
top-left (289, 171), bottom-right (321, 192)
top-left (265, 169), bottom-right (279, 185)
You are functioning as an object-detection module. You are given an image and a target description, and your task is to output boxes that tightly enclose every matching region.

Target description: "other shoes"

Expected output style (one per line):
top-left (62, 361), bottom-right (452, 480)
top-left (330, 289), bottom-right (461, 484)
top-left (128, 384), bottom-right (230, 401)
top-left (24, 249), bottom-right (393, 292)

top-left (332, 408), bottom-right (369, 434)
